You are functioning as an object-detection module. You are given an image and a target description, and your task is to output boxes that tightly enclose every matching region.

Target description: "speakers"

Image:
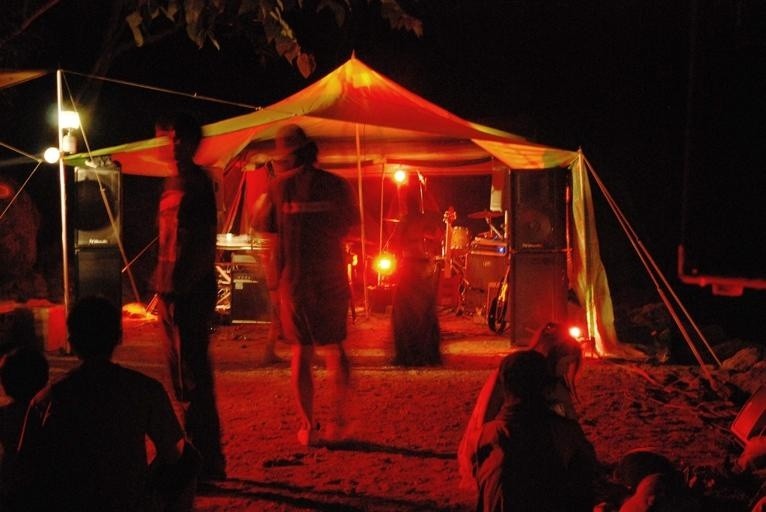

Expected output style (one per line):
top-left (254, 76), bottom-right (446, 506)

top-left (76, 166), bottom-right (121, 248)
top-left (73, 251), bottom-right (121, 339)
top-left (509, 169), bottom-right (565, 251)
top-left (509, 253), bottom-right (568, 343)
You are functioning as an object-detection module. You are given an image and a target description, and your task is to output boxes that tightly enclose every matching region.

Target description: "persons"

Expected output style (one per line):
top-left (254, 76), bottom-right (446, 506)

top-left (1, 110), bottom-right (228, 510)
top-left (457, 319), bottom-right (666, 511)
top-left (387, 187), bottom-right (445, 368)
top-left (237, 123), bottom-right (362, 447)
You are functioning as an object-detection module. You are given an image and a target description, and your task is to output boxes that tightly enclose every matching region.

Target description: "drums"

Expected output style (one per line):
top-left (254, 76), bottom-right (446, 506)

top-left (449, 225), bottom-right (469, 253)
top-left (471, 236), bottom-right (506, 286)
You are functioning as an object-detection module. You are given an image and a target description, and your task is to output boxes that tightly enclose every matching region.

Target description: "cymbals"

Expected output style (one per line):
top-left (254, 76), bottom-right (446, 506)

top-left (466, 210), bottom-right (501, 219)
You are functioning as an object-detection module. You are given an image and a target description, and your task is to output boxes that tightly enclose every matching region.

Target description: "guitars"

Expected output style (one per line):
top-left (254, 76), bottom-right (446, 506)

top-left (490, 247), bottom-right (513, 333)
top-left (433, 209), bottom-right (463, 314)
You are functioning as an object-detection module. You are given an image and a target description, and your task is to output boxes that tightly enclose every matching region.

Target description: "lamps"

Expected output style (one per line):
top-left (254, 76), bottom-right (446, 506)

top-left (42, 110), bottom-right (80, 164)
